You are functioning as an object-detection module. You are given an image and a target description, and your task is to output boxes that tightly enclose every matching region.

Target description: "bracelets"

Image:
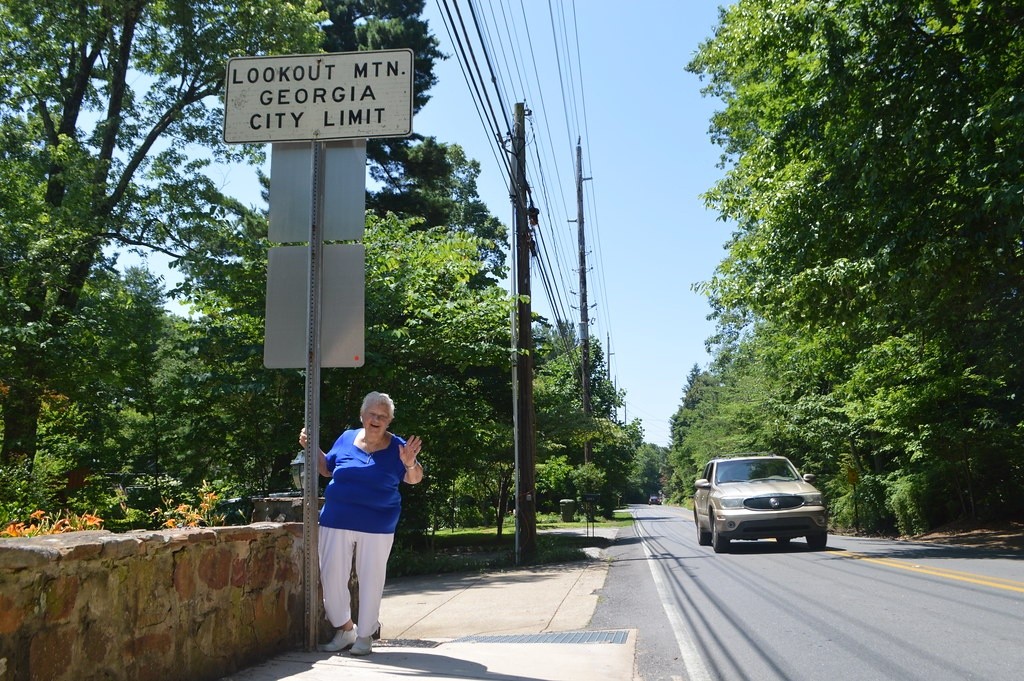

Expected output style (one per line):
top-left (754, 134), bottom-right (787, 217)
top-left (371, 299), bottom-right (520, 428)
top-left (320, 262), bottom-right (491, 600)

top-left (404, 459), bottom-right (417, 470)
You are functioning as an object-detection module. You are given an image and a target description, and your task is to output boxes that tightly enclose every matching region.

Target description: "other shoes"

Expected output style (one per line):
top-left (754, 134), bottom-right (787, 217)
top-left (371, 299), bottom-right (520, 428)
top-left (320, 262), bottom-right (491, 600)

top-left (323, 624), bottom-right (358, 651)
top-left (351, 636), bottom-right (372, 655)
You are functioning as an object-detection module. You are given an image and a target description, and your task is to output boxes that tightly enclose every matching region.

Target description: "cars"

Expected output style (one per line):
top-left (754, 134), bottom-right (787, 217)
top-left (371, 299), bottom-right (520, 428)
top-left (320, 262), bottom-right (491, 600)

top-left (648, 496), bottom-right (660, 505)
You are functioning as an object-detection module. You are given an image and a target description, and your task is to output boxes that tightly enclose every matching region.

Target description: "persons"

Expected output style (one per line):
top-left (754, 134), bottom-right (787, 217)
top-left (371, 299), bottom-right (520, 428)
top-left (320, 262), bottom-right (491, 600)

top-left (298, 391), bottom-right (425, 656)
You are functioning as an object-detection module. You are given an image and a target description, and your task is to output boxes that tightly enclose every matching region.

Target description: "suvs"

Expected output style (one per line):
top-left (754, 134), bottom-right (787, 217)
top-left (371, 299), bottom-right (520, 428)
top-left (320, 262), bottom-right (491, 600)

top-left (693, 455), bottom-right (827, 553)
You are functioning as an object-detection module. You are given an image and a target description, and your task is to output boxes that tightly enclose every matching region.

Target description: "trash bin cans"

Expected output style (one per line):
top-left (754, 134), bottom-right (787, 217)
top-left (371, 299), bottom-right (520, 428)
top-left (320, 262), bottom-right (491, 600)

top-left (252, 492), bottom-right (328, 530)
top-left (560, 499), bottom-right (576, 522)
